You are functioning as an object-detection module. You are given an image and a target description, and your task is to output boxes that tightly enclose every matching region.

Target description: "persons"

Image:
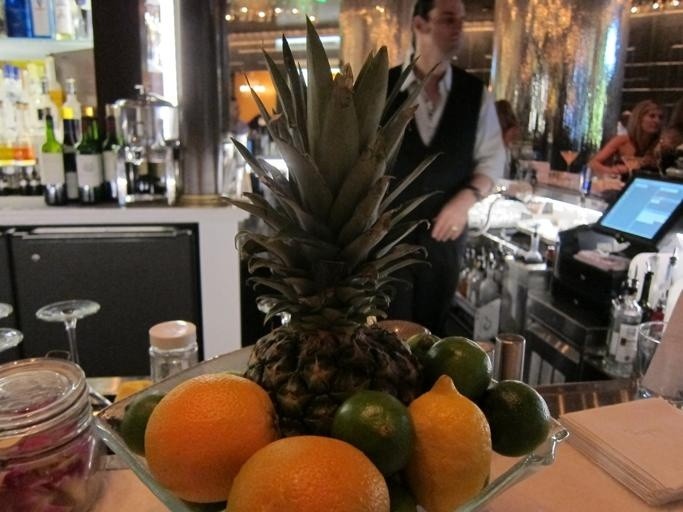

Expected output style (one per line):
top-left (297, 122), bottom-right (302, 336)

top-left (612, 110), bottom-right (636, 167)
top-left (493, 98), bottom-right (525, 180)
top-left (375, 0), bottom-right (509, 336)
top-left (227, 96), bottom-right (245, 135)
top-left (588, 100), bottom-right (672, 181)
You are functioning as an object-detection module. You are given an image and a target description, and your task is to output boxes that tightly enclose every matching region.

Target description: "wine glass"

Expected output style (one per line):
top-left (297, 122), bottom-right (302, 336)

top-left (0, 300), bottom-right (111, 418)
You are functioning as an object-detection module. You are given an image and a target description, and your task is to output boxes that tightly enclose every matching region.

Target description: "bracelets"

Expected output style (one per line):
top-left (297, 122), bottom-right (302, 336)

top-left (464, 184), bottom-right (485, 203)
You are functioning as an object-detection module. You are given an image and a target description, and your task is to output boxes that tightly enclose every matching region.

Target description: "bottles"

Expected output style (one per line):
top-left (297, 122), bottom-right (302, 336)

top-left (0, 1), bottom-right (183, 206)
top-left (487, 331), bottom-right (527, 389)
top-left (604, 275), bottom-right (643, 378)
top-left (443, 228), bottom-right (543, 304)
top-left (0, 353), bottom-right (103, 512)
top-left (147, 318), bottom-right (202, 380)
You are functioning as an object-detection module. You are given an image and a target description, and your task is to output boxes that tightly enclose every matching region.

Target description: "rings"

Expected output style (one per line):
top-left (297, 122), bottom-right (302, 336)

top-left (449, 226), bottom-right (458, 233)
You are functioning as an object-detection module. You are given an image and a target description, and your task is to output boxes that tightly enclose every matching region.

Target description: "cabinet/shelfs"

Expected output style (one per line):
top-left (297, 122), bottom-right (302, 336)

top-left (5, 223), bottom-right (200, 376)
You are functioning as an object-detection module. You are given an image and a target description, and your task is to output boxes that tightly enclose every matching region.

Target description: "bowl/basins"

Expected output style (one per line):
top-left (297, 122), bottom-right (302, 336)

top-left (88, 317), bottom-right (570, 512)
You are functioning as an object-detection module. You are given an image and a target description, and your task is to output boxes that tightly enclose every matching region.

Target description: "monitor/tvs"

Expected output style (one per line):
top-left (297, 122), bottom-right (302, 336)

top-left (595, 173), bottom-right (683, 246)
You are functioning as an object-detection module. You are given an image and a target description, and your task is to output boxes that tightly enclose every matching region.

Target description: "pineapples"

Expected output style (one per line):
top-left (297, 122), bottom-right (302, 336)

top-left (244, 323), bottom-right (424, 437)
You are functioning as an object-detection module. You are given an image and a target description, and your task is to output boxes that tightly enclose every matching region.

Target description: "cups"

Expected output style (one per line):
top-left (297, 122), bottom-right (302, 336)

top-left (644, 321), bottom-right (667, 375)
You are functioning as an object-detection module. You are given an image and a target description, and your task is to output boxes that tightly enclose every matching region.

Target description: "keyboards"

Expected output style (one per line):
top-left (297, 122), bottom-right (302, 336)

top-left (573, 250), bottom-right (631, 271)
